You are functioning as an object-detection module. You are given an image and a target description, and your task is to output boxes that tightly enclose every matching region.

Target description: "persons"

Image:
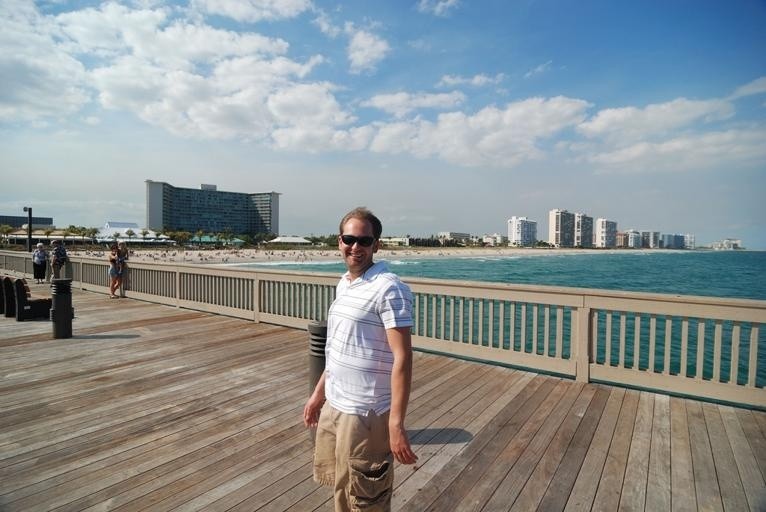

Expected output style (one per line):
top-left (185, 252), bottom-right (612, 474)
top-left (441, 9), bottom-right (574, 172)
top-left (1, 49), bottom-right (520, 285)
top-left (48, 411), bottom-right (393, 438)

top-left (109, 245), bottom-right (125, 297)
top-left (303, 207), bottom-right (418, 511)
top-left (117, 240), bottom-right (129, 275)
top-left (49, 240), bottom-right (66, 283)
top-left (32, 243), bottom-right (50, 284)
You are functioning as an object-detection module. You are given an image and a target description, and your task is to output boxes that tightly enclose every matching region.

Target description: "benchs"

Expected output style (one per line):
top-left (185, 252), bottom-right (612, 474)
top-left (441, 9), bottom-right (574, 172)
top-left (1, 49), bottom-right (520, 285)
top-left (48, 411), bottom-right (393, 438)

top-left (0, 274), bottom-right (51, 322)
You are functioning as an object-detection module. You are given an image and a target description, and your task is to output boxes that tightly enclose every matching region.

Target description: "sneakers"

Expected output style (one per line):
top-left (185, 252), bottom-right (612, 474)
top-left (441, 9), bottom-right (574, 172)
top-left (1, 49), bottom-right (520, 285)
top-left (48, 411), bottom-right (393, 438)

top-left (110, 295), bottom-right (119, 298)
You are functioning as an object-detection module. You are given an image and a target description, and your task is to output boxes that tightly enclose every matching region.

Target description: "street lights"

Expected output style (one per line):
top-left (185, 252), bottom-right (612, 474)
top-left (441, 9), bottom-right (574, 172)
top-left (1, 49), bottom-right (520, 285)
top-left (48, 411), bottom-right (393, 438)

top-left (21, 206), bottom-right (33, 251)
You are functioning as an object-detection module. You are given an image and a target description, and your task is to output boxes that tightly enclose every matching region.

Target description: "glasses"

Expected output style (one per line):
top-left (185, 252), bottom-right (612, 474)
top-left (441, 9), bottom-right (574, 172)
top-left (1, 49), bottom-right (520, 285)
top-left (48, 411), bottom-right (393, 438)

top-left (342, 233), bottom-right (375, 247)
